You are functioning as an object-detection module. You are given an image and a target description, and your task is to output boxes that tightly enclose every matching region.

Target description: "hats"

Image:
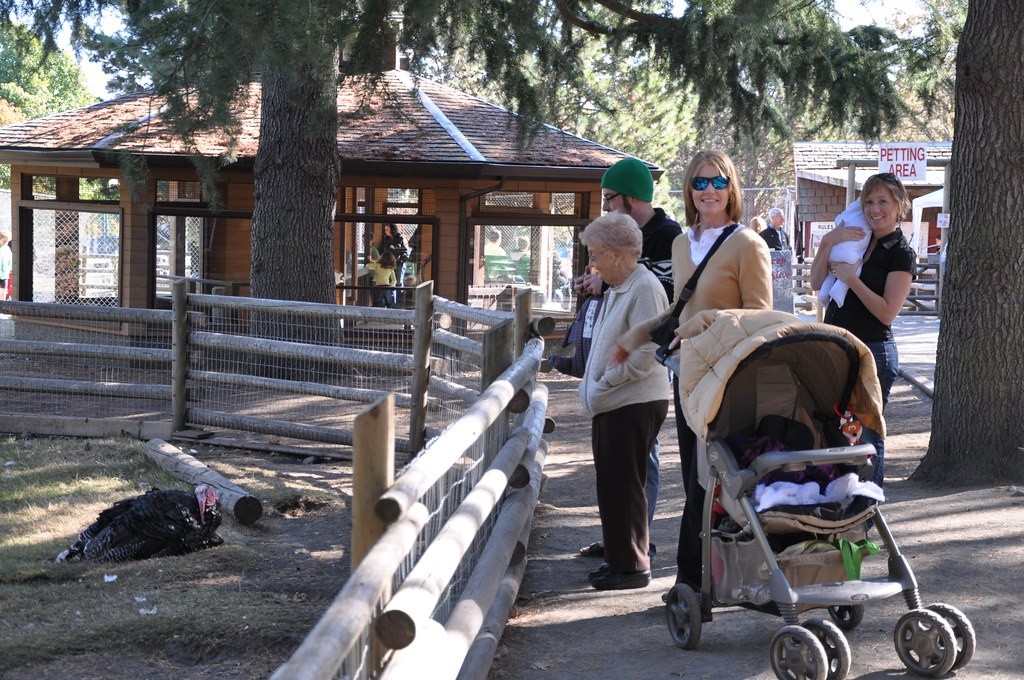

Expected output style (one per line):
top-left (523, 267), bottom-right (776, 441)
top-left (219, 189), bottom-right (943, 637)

top-left (601, 157), bottom-right (653, 202)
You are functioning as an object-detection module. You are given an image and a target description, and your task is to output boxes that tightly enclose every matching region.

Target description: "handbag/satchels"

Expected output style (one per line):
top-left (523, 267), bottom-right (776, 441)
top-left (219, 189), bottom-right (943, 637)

top-left (648, 313), bottom-right (679, 347)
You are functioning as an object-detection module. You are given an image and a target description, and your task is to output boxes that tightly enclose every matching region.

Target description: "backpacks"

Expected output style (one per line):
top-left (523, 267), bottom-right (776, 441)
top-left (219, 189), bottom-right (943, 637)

top-left (551, 296), bottom-right (608, 379)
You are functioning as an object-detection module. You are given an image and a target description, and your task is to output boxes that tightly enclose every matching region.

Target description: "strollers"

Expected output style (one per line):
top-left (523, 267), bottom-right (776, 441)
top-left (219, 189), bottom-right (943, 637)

top-left (654, 310), bottom-right (976, 680)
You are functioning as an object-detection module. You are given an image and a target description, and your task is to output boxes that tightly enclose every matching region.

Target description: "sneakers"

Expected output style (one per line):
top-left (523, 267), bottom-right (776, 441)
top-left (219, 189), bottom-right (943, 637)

top-left (588, 562), bottom-right (651, 589)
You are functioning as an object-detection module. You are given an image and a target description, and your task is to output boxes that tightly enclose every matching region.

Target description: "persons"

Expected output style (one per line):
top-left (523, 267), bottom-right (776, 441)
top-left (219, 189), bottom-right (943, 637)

top-left (580, 212), bottom-right (670, 589)
top-left (484, 230), bottom-right (507, 283)
top-left (0, 231), bottom-right (12, 302)
top-left (751, 217), bottom-right (767, 235)
top-left (574, 157), bottom-right (683, 568)
top-left (810, 173), bottom-right (917, 525)
top-left (511, 237), bottom-right (530, 264)
top-left (378, 224), bottom-right (407, 306)
top-left (366, 251), bottom-right (398, 309)
top-left (759, 209), bottom-right (793, 256)
top-left (363, 229), bottom-right (380, 301)
top-left (189, 240), bottom-right (199, 277)
top-left (606, 150), bottom-right (773, 604)
top-left (936, 235), bottom-right (941, 254)
top-left (819, 197), bottom-right (872, 307)
top-left (552, 244), bottom-right (568, 300)
top-left (408, 213), bottom-right (419, 278)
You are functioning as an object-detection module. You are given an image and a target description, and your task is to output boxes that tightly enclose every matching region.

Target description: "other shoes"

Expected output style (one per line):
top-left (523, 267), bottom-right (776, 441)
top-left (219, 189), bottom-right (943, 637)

top-left (579, 541), bottom-right (604, 556)
top-left (661, 592), bottom-right (668, 603)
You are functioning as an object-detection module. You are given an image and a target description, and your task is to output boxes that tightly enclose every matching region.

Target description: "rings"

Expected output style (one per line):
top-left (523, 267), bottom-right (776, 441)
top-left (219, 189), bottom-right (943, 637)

top-left (833, 270), bottom-right (835, 274)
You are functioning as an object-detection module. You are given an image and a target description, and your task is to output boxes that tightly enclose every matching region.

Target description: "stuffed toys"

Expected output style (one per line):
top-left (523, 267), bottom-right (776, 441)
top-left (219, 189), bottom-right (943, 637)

top-left (834, 403), bottom-right (862, 445)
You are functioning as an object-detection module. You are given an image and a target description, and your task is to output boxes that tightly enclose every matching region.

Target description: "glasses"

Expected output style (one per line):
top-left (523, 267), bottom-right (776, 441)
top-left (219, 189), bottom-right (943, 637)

top-left (587, 248), bottom-right (609, 261)
top-left (868, 172), bottom-right (898, 183)
top-left (603, 192), bottom-right (621, 203)
top-left (691, 175), bottom-right (730, 191)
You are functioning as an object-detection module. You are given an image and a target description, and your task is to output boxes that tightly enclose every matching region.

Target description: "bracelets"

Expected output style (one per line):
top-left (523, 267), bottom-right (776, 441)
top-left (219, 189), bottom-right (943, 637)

top-left (601, 376), bottom-right (610, 387)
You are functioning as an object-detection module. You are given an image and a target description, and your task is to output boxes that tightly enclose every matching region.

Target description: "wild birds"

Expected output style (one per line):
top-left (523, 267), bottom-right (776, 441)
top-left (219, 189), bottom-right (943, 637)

top-left (56, 484), bottom-right (225, 561)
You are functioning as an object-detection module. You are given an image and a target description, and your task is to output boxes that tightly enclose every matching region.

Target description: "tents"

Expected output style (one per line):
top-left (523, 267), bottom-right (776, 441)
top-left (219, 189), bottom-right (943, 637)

top-left (913, 189), bottom-right (943, 275)
